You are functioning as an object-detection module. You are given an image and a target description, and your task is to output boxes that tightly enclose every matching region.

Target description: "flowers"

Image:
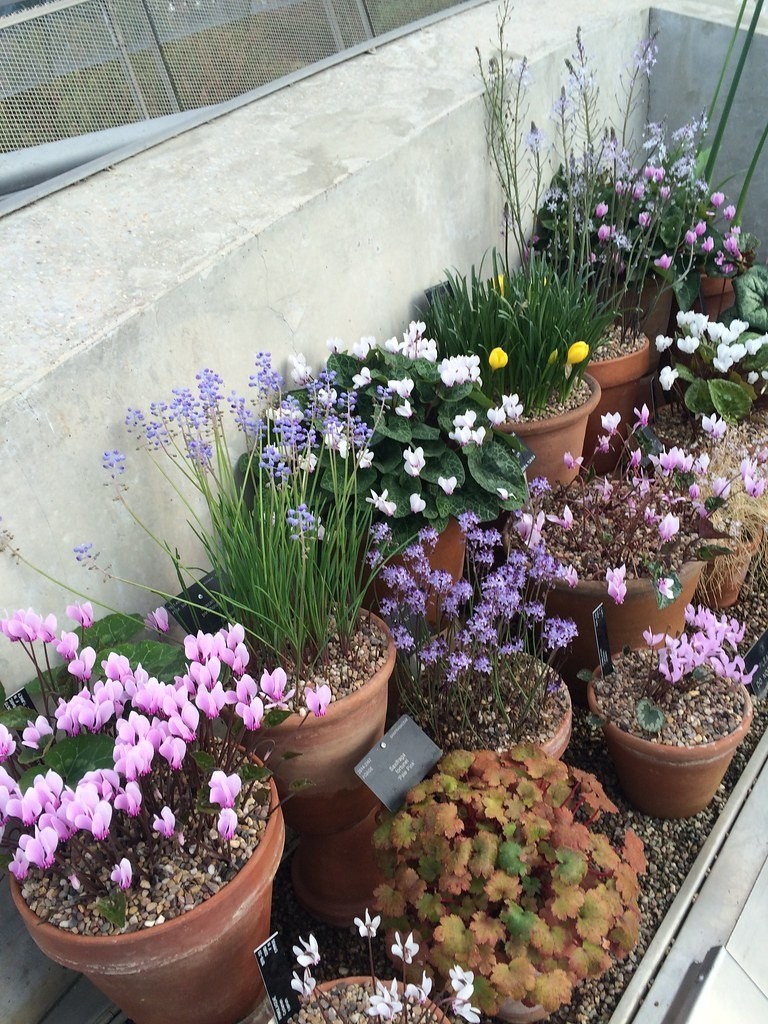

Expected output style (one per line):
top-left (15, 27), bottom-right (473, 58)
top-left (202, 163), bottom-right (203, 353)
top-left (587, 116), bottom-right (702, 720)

top-left (542, 401), bottom-right (768, 610)
top-left (476, 0), bottom-right (708, 346)
top-left (0, 600), bottom-right (332, 929)
top-left (364, 477), bottom-right (578, 754)
top-left (291, 908), bottom-right (481, 1024)
top-left (239, 319), bottom-right (527, 556)
top-left (0, 351), bottom-right (419, 708)
top-left (635, 603), bottom-right (758, 731)
top-left (411, 246), bottom-right (638, 417)
top-left (655, 310), bottom-right (768, 443)
top-left (523, 146), bottom-right (745, 315)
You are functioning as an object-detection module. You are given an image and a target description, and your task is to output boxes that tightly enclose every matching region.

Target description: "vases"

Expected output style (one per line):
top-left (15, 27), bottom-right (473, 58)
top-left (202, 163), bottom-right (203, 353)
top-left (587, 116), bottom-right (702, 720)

top-left (505, 479), bottom-right (704, 705)
top-left (591, 277), bottom-right (675, 377)
top-left (332, 515), bottom-right (466, 636)
top-left (637, 371), bottom-right (666, 418)
top-left (587, 647), bottom-right (753, 820)
top-left (9, 736), bottom-right (285, 1024)
top-left (585, 324), bottom-right (650, 474)
top-left (492, 368), bottom-right (601, 488)
top-left (219, 601), bottom-right (396, 835)
top-left (385, 616), bottom-right (464, 732)
top-left (291, 803), bottom-right (396, 928)
top-left (397, 652), bottom-right (571, 760)
top-left (269, 976), bottom-right (451, 1024)
top-left (628, 401), bottom-right (768, 492)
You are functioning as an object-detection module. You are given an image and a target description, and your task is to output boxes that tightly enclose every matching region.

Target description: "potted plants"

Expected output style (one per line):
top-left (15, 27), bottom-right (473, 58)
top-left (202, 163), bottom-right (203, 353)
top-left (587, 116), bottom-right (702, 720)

top-left (686, 424), bottom-right (768, 608)
top-left (677, 0), bottom-right (768, 323)
top-left (369, 743), bottom-right (647, 1024)
